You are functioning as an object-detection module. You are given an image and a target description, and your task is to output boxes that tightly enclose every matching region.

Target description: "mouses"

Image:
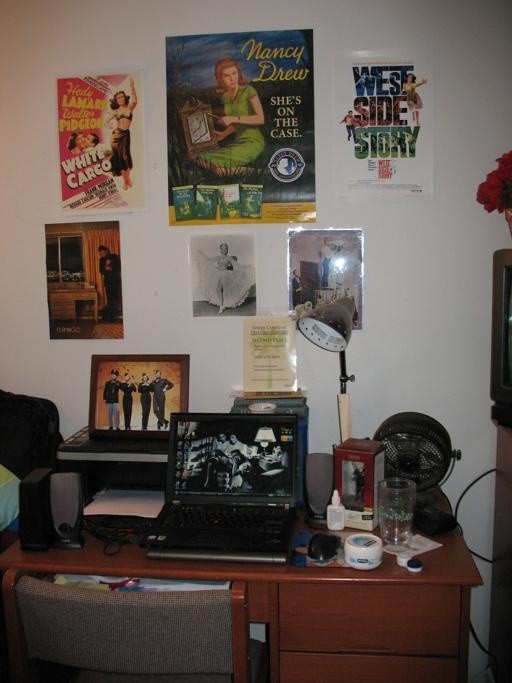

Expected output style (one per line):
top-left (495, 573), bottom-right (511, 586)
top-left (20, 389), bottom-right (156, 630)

top-left (307, 533), bottom-right (341, 562)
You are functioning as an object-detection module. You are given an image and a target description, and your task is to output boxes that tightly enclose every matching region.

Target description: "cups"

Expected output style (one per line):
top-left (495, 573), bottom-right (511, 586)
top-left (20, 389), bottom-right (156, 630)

top-left (376, 477), bottom-right (417, 555)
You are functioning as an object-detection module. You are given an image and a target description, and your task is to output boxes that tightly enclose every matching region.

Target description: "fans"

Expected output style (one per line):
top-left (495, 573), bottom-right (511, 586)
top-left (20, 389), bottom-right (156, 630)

top-left (363, 409), bottom-right (459, 535)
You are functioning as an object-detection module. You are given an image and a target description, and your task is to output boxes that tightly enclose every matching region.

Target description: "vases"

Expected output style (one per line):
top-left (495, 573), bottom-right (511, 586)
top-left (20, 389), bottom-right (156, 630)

top-left (500, 208), bottom-right (511, 238)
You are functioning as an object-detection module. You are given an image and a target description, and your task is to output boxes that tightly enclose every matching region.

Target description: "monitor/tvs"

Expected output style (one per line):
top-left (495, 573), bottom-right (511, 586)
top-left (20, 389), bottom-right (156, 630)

top-left (490, 248), bottom-right (512, 427)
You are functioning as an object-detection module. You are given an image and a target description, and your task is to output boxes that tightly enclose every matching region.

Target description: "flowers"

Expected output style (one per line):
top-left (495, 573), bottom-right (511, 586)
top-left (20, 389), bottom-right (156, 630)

top-left (474, 150), bottom-right (511, 211)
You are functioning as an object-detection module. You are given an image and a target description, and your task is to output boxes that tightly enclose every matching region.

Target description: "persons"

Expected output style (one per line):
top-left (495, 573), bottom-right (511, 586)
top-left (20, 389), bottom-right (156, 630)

top-left (196, 242), bottom-right (256, 314)
top-left (105, 77), bottom-right (136, 194)
top-left (293, 234), bottom-right (344, 308)
top-left (183, 57), bottom-right (272, 177)
top-left (338, 110), bottom-right (357, 143)
top-left (398, 73), bottom-right (428, 125)
top-left (103, 367), bottom-right (175, 431)
top-left (68, 133), bottom-right (99, 155)
top-left (353, 462), bottom-right (366, 504)
top-left (98, 244), bottom-right (122, 323)
top-left (202, 432), bottom-right (259, 497)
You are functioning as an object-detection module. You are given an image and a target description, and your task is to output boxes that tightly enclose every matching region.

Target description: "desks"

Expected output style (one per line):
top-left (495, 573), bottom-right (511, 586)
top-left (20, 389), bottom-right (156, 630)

top-left (1, 490), bottom-right (484, 681)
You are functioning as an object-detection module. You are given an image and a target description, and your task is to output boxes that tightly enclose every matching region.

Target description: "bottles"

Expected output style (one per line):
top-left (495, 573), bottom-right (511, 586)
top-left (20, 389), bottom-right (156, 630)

top-left (325, 490), bottom-right (346, 532)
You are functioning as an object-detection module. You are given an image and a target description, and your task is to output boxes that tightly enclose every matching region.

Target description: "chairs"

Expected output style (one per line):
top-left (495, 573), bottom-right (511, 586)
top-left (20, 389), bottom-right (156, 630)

top-left (2, 566), bottom-right (270, 681)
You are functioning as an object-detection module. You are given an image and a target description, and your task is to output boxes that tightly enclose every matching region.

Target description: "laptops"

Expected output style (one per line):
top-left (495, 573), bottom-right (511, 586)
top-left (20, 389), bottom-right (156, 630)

top-left (147, 411), bottom-right (296, 565)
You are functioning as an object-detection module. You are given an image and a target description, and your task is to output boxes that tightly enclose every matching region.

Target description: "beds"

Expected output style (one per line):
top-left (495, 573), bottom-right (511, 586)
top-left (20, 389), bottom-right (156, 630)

top-left (1, 385), bottom-right (65, 555)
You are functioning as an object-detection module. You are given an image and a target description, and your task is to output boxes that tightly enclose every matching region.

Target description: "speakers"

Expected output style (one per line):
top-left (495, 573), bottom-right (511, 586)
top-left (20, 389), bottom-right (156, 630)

top-left (50, 473), bottom-right (84, 549)
top-left (305, 452), bottom-right (335, 530)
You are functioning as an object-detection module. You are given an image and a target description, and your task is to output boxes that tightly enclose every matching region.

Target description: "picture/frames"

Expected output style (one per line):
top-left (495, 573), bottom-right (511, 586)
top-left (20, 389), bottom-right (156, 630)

top-left (285, 225), bottom-right (366, 333)
top-left (87, 351), bottom-right (191, 438)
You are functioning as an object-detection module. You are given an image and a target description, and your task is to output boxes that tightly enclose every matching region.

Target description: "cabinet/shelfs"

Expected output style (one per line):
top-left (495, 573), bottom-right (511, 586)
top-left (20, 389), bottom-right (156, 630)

top-left (486, 403), bottom-right (512, 681)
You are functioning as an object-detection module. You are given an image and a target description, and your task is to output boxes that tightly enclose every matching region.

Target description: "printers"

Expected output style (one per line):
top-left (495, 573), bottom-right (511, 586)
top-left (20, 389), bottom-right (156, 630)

top-left (56, 420), bottom-right (176, 529)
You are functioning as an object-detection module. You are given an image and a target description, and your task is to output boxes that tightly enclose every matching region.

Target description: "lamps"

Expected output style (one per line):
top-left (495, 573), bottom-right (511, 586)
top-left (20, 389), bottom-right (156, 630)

top-left (295, 297), bottom-right (359, 444)
